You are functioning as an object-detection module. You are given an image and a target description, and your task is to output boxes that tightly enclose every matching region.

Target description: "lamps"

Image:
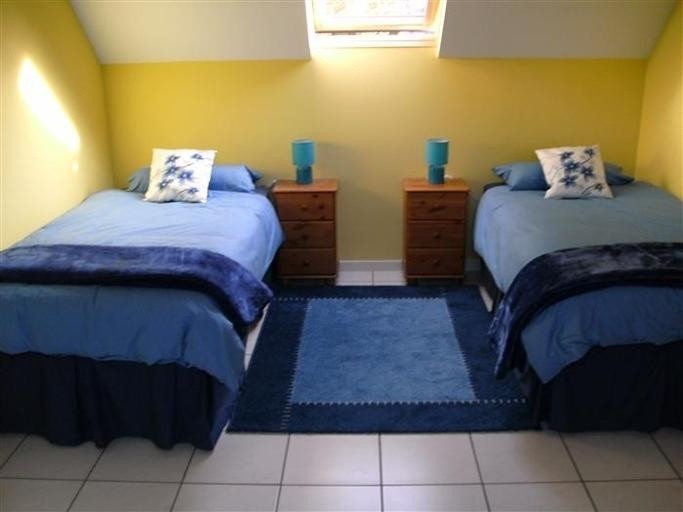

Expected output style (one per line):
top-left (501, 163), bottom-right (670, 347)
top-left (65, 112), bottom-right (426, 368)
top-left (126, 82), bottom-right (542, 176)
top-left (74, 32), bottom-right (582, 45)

top-left (292, 139), bottom-right (315, 185)
top-left (427, 139), bottom-right (448, 184)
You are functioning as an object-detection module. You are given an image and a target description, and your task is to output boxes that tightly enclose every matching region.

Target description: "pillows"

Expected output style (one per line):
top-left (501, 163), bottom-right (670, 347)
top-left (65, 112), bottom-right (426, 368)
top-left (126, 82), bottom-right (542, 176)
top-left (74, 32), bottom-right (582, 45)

top-left (534, 144), bottom-right (614, 199)
top-left (491, 161), bottom-right (634, 191)
top-left (126, 164), bottom-right (263, 194)
top-left (142, 149), bottom-right (218, 204)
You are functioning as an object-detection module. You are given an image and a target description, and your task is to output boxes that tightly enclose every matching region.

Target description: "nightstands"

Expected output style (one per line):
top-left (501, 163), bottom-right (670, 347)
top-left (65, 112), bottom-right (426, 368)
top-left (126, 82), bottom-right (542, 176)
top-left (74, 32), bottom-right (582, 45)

top-left (271, 179), bottom-right (340, 286)
top-left (402, 177), bottom-right (471, 286)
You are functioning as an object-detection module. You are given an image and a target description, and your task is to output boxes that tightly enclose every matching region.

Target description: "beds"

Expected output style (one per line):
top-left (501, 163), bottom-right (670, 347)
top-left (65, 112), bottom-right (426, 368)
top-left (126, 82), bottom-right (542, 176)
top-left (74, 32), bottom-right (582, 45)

top-left (0, 186), bottom-right (286, 450)
top-left (472, 182), bottom-right (683, 433)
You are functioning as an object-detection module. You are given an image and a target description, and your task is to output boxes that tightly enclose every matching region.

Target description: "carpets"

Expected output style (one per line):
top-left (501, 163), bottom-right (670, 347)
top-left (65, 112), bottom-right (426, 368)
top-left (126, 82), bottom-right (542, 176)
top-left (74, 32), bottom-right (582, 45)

top-left (226, 286), bottom-right (543, 433)
